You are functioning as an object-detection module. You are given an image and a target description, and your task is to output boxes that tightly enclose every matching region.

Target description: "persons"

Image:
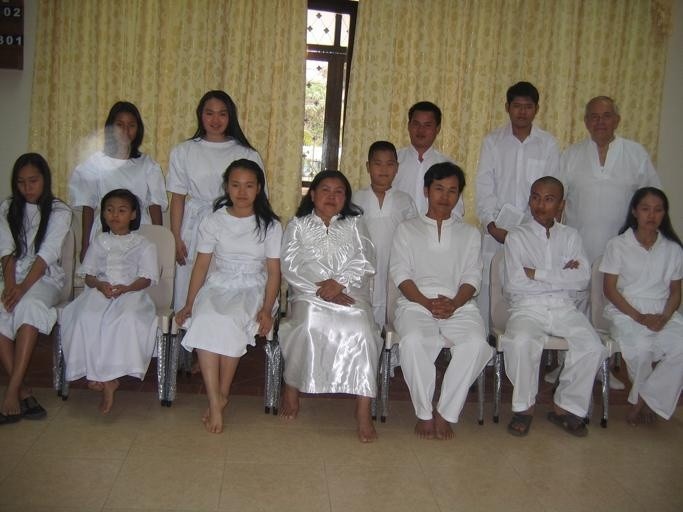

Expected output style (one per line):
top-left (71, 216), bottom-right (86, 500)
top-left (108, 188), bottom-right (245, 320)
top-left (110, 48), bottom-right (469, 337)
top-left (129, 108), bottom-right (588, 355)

top-left (474, 81), bottom-right (561, 345)
top-left (598, 187), bottom-right (682, 426)
top-left (59, 189), bottom-right (161, 414)
top-left (389, 162), bottom-right (491, 439)
top-left (351, 141), bottom-right (419, 326)
top-left (174, 158), bottom-right (283, 434)
top-left (0, 153), bottom-right (72, 425)
top-left (543, 95), bottom-right (664, 390)
top-left (71, 101), bottom-right (168, 264)
top-left (503, 175), bottom-right (600, 437)
top-left (279, 170), bottom-right (380, 443)
top-left (389, 102), bottom-right (466, 218)
top-left (164, 90), bottom-right (270, 378)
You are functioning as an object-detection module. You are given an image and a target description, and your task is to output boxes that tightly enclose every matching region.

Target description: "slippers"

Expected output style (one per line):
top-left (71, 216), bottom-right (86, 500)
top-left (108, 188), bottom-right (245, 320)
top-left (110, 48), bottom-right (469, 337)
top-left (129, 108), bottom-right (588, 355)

top-left (0, 396), bottom-right (46, 424)
top-left (547, 411), bottom-right (587, 437)
top-left (507, 414), bottom-right (533, 437)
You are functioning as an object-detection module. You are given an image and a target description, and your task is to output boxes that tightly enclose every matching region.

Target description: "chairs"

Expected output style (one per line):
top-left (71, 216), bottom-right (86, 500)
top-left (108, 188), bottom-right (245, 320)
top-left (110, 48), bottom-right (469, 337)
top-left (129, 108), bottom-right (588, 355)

top-left (167, 261), bottom-right (272, 415)
top-left (50, 227), bottom-right (75, 399)
top-left (59, 226), bottom-right (175, 407)
top-left (382, 234), bottom-right (491, 427)
top-left (590, 254), bottom-right (621, 426)
top-left (274, 221), bottom-right (387, 422)
top-left (491, 245), bottom-right (611, 429)
